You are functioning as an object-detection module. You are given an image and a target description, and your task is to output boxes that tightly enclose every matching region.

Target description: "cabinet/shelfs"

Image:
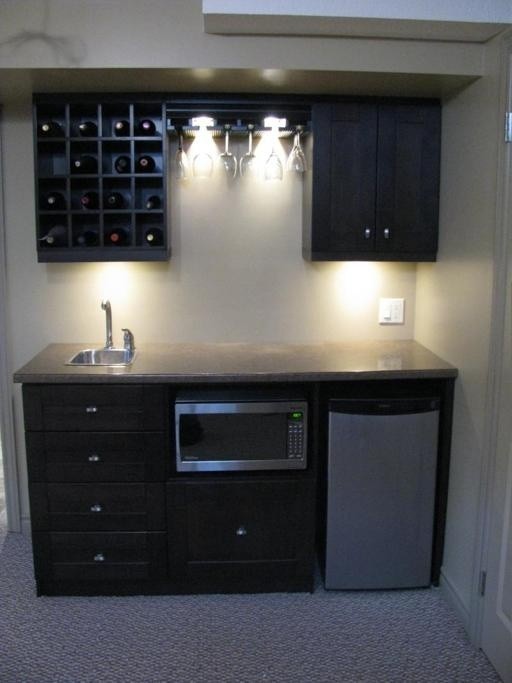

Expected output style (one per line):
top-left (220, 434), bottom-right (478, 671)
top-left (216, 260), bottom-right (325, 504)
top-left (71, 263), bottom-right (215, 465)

top-left (310, 95), bottom-right (440, 262)
top-left (166, 476), bottom-right (318, 594)
top-left (21, 382), bottom-right (168, 597)
top-left (31, 90), bottom-right (169, 260)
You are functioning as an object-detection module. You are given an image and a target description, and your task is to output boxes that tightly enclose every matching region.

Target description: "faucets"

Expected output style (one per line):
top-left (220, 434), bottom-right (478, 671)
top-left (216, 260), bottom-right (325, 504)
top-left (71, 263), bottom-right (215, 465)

top-left (96, 295), bottom-right (116, 349)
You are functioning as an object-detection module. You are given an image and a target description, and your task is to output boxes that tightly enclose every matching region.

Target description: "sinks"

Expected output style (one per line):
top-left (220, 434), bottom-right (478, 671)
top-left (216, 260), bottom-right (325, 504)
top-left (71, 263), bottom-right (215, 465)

top-left (64, 346), bottom-right (131, 371)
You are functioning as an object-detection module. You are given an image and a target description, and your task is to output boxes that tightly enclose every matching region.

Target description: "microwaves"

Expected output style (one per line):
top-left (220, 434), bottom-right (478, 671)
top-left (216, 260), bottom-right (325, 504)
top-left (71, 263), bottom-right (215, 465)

top-left (173, 397), bottom-right (308, 473)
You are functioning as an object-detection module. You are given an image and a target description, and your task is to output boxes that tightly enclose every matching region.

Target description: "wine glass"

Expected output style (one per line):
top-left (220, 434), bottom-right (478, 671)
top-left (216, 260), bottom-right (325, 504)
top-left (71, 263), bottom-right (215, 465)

top-left (171, 123), bottom-right (306, 181)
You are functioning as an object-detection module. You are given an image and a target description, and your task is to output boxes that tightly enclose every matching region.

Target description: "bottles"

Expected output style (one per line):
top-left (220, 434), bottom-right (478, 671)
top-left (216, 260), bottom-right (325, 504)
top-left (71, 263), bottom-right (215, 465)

top-left (40, 119), bottom-right (163, 246)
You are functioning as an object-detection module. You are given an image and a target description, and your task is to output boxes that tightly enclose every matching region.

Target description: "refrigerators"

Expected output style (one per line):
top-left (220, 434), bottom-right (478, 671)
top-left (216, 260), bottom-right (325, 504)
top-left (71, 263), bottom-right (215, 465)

top-left (325, 398), bottom-right (439, 591)
